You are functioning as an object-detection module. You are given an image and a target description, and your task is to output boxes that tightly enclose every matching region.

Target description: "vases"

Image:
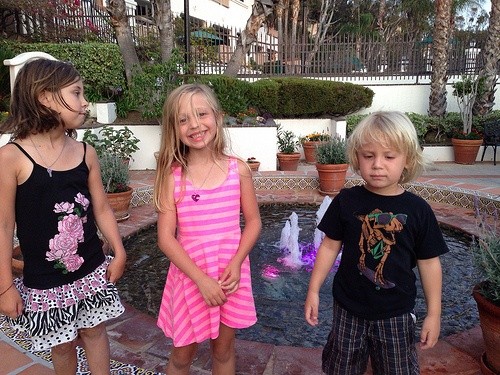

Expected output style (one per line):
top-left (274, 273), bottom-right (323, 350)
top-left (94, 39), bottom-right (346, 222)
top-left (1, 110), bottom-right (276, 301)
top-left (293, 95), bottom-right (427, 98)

top-left (451, 138), bottom-right (483, 165)
top-left (277, 153), bottom-right (301, 171)
top-left (472, 281), bottom-right (500, 373)
top-left (106, 186), bottom-right (133, 219)
top-left (302, 141), bottom-right (320, 165)
top-left (248, 161), bottom-right (259, 171)
top-left (315, 162), bottom-right (349, 192)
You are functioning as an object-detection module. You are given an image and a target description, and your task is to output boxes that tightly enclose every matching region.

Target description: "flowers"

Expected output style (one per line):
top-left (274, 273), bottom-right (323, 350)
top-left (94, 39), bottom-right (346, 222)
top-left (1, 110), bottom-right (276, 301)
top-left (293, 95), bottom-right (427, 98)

top-left (82, 125), bottom-right (140, 193)
top-left (247, 157), bottom-right (255, 163)
top-left (453, 70), bottom-right (484, 140)
top-left (276, 126), bottom-right (347, 164)
top-left (468, 199), bottom-right (500, 306)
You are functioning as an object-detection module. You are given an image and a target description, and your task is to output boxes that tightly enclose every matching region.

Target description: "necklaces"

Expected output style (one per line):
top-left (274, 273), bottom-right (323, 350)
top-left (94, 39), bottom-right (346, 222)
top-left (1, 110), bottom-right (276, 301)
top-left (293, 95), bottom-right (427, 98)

top-left (191, 160), bottom-right (215, 202)
top-left (28, 133), bottom-right (66, 178)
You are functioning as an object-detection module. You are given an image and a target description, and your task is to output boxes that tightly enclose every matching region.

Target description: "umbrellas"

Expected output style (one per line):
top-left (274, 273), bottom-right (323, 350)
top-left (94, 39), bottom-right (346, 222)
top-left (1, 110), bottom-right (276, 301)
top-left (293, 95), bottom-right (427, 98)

top-left (414, 33), bottom-right (459, 44)
top-left (177, 29), bottom-right (224, 45)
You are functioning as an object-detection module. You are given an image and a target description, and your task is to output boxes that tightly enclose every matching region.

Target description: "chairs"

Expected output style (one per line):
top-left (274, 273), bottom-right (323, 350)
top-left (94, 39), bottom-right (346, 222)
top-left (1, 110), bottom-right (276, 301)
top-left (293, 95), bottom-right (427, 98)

top-left (482, 119), bottom-right (500, 166)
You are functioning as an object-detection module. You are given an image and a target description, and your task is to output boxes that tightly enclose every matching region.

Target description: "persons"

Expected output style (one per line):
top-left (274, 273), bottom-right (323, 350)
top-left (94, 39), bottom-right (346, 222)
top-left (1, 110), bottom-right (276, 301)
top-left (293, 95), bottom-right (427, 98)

top-left (153, 84), bottom-right (263, 375)
top-left (0, 58), bottom-right (126, 375)
top-left (305, 111), bottom-right (449, 375)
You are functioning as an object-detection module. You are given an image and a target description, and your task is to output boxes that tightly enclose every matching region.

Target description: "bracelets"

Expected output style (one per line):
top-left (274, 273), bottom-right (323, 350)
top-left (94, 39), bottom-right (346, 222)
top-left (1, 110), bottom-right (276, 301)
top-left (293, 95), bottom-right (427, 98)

top-left (0, 284), bottom-right (12, 296)
